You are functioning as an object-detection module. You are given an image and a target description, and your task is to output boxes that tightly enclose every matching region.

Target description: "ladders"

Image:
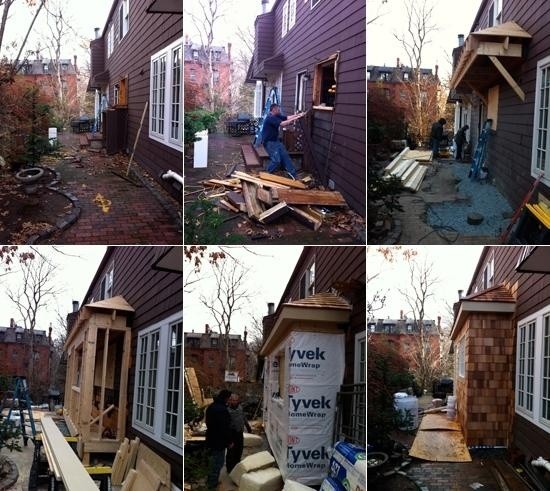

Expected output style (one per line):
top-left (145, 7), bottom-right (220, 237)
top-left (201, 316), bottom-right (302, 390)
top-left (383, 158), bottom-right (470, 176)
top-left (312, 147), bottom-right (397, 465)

top-left (93, 95), bottom-right (110, 136)
top-left (468, 119), bottom-right (492, 181)
top-left (254, 86), bottom-right (284, 146)
top-left (0, 376), bottom-right (36, 436)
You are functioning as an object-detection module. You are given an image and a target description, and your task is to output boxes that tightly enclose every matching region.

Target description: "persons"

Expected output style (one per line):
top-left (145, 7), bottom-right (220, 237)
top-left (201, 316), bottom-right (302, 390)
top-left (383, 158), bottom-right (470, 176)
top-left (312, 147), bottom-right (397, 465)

top-left (454, 124), bottom-right (470, 161)
top-left (226, 394), bottom-right (253, 474)
top-left (261, 104), bottom-right (308, 179)
top-left (205, 389), bottom-right (235, 488)
top-left (430, 118), bottom-right (447, 158)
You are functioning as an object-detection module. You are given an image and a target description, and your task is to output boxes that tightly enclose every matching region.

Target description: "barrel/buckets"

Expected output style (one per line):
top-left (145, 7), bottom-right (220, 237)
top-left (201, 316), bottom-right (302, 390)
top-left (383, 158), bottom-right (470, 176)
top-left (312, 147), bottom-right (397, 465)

top-left (393, 395), bottom-right (419, 431)
top-left (446, 396), bottom-right (457, 417)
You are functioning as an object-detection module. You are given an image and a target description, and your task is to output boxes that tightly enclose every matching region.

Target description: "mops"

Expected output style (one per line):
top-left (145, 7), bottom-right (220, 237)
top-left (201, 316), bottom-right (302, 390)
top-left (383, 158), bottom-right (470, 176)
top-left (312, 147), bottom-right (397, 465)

top-left (111, 100), bottom-right (148, 187)
